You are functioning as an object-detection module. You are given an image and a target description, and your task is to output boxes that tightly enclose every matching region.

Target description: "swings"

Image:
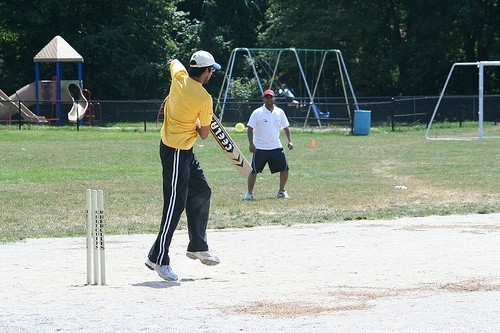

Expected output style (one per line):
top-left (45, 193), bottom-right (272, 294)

top-left (250, 50), bottom-right (331, 120)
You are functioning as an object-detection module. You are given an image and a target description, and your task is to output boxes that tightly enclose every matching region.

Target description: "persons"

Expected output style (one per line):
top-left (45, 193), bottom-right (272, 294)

top-left (275, 84), bottom-right (294, 102)
top-left (246, 90), bottom-right (293, 199)
top-left (145, 51), bottom-right (222, 281)
top-left (72, 88), bottom-right (79, 100)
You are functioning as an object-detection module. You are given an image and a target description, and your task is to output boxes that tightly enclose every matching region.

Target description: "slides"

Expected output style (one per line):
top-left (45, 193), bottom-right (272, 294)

top-left (60, 82), bottom-right (88, 123)
top-left (0, 81), bottom-right (36, 120)
top-left (0, 89), bottom-right (48, 123)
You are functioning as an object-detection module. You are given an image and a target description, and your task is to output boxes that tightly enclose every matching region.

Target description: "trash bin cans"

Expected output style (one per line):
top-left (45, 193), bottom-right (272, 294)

top-left (353, 110), bottom-right (371, 134)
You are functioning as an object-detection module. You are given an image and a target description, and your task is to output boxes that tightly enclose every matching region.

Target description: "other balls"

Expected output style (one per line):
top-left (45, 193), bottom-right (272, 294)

top-left (235, 123), bottom-right (244, 132)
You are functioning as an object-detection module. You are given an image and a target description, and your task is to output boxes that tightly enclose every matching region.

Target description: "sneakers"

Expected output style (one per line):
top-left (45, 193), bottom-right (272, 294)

top-left (278, 190), bottom-right (289, 198)
top-left (187, 250), bottom-right (221, 265)
top-left (145, 258), bottom-right (177, 281)
top-left (246, 192), bottom-right (253, 199)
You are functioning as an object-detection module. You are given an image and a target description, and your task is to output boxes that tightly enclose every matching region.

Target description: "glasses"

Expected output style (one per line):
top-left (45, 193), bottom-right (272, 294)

top-left (208, 68), bottom-right (215, 72)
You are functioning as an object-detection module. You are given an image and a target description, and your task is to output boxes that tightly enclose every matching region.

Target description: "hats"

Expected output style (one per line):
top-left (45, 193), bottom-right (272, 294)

top-left (190, 51), bottom-right (221, 70)
top-left (263, 90), bottom-right (274, 97)
